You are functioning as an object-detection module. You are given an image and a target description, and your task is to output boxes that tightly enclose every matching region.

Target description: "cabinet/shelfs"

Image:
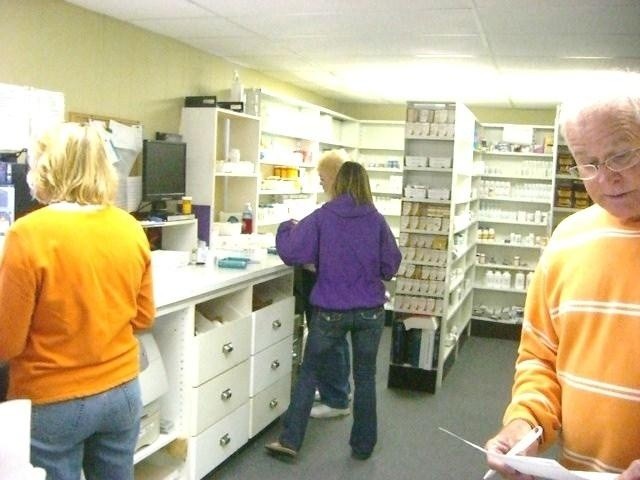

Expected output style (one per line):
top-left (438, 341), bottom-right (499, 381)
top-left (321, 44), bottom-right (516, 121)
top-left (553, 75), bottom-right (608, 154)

top-left (179, 107), bottom-right (260, 237)
top-left (192, 263), bottom-right (295, 480)
top-left (243, 87), bottom-right (359, 236)
top-left (471, 124), bottom-right (587, 339)
top-left (0, 299), bottom-right (192, 480)
top-left (390, 101), bottom-right (479, 396)
top-left (359, 118), bottom-right (405, 325)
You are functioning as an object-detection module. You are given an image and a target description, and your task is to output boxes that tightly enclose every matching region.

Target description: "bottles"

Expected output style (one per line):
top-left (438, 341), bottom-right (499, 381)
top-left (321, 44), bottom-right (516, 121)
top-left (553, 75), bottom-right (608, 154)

top-left (259, 203), bottom-right (274, 224)
top-left (521, 158), bottom-right (554, 178)
top-left (195, 241), bottom-right (208, 265)
top-left (242, 202), bottom-right (252, 234)
top-left (476, 180), bottom-right (552, 290)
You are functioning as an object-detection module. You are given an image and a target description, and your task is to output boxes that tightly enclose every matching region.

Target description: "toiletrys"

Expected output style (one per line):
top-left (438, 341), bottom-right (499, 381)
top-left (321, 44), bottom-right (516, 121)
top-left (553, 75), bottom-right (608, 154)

top-left (213, 256), bottom-right (247, 268)
top-left (241, 202), bottom-right (253, 233)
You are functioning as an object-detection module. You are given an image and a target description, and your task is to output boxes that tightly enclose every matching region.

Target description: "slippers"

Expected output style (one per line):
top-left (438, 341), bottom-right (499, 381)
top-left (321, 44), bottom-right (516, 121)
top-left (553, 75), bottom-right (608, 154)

top-left (265, 442), bottom-right (296, 456)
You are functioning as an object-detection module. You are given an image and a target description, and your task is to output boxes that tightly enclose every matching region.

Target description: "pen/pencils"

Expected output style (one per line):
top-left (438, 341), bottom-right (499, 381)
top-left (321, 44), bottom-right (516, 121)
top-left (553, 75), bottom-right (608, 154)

top-left (482, 426), bottom-right (541, 480)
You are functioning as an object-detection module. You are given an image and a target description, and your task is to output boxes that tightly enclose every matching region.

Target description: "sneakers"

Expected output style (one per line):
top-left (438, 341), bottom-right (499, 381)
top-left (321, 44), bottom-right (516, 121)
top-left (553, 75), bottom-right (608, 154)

top-left (310, 404), bottom-right (350, 418)
top-left (315, 390), bottom-right (353, 402)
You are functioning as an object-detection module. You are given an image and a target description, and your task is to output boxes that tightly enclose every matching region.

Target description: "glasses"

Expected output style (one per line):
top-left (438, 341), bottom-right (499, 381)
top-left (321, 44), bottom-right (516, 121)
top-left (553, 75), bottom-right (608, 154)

top-left (565, 148), bottom-right (640, 181)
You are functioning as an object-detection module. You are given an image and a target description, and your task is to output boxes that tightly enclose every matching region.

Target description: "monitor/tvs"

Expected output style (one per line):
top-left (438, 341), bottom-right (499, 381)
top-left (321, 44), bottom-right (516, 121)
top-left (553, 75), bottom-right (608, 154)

top-left (143, 140), bottom-right (187, 202)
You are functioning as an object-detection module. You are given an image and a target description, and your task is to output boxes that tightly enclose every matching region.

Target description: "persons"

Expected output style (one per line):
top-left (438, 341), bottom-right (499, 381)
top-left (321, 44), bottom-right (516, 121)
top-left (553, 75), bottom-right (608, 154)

top-left (485, 89), bottom-right (639, 479)
top-left (300, 147), bottom-right (353, 421)
top-left (265, 160), bottom-right (402, 460)
top-left (0, 120), bottom-right (156, 479)
